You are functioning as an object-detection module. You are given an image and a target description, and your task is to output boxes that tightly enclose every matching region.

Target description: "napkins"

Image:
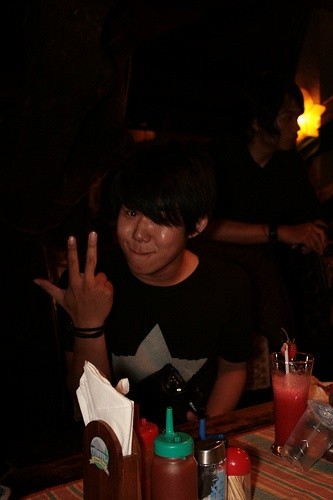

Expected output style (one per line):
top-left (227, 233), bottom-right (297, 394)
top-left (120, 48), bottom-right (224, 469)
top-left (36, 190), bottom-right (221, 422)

top-left (75, 362), bottom-right (135, 458)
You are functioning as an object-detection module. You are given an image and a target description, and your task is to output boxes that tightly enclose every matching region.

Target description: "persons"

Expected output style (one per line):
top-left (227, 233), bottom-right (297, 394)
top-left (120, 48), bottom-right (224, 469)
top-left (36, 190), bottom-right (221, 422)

top-left (202, 77), bottom-right (327, 257)
top-left (32, 141), bottom-right (252, 428)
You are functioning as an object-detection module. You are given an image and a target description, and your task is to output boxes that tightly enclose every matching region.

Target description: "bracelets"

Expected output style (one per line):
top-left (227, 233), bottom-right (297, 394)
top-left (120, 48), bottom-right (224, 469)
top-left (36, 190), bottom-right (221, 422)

top-left (72, 325), bottom-right (105, 338)
top-left (269, 225), bottom-right (277, 242)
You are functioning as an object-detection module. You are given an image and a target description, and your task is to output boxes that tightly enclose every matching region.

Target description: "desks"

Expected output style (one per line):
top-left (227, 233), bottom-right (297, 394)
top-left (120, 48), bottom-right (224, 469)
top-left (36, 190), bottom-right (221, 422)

top-left (19, 399), bottom-right (333, 499)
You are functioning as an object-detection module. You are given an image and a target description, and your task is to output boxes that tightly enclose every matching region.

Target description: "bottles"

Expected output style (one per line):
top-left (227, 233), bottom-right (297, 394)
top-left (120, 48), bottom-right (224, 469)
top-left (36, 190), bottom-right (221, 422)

top-left (134, 407), bottom-right (228, 500)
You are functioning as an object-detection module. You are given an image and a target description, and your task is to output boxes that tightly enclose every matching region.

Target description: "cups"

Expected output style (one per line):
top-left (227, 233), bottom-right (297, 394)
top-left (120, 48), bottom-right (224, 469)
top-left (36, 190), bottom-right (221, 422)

top-left (280, 401), bottom-right (333, 472)
top-left (269, 351), bottom-right (314, 458)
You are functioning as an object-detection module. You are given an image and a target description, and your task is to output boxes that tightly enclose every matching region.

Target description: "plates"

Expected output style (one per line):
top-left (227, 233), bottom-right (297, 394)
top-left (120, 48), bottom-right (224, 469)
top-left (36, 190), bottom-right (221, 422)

top-left (306, 380), bottom-right (332, 458)
